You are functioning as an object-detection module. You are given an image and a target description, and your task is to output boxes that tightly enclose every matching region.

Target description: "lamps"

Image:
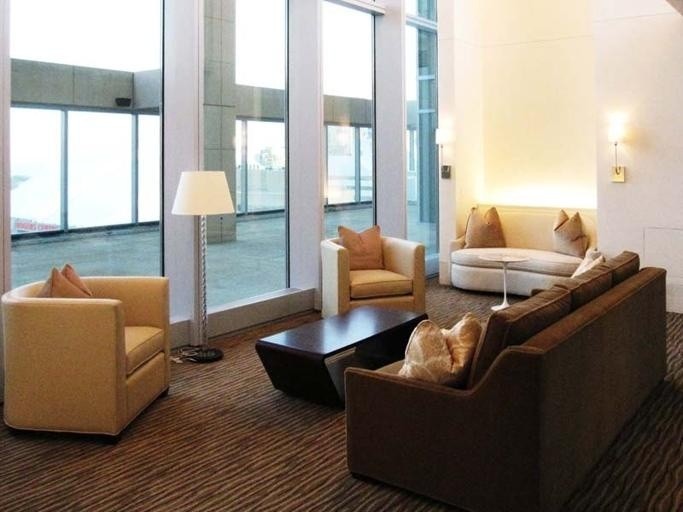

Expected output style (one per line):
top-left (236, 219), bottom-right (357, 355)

top-left (171, 171), bottom-right (234, 363)
top-left (434, 128), bottom-right (451, 180)
top-left (607, 119), bottom-right (626, 184)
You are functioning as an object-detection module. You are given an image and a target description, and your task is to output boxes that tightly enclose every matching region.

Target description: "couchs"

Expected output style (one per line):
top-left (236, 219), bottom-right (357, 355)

top-left (321, 235), bottom-right (427, 316)
top-left (1, 275), bottom-right (171, 435)
top-left (438, 204), bottom-right (598, 296)
top-left (346, 250), bottom-right (667, 509)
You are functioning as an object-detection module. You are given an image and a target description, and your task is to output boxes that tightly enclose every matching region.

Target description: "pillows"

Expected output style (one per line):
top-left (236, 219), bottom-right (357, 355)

top-left (37, 265), bottom-right (92, 297)
top-left (338, 227), bottom-right (385, 266)
top-left (398, 312), bottom-right (484, 385)
top-left (572, 248), bottom-right (607, 278)
top-left (464, 206), bottom-right (506, 248)
top-left (553, 209), bottom-right (589, 257)
